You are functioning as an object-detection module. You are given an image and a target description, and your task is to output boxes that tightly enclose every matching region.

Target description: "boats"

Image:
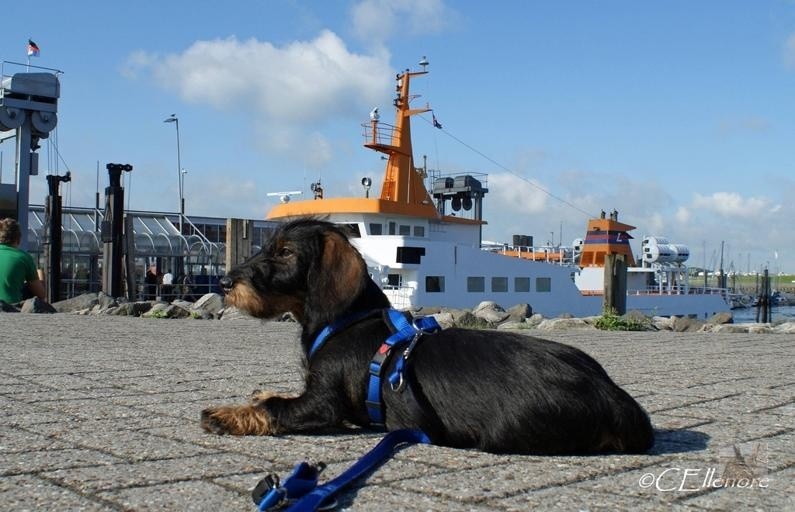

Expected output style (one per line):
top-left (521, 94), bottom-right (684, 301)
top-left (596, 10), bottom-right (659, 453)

top-left (266, 56), bottom-right (734, 323)
top-left (728, 291), bottom-right (795, 310)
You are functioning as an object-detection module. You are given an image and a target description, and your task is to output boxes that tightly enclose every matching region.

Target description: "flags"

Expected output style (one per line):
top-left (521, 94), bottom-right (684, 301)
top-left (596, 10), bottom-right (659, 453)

top-left (27, 37), bottom-right (40, 59)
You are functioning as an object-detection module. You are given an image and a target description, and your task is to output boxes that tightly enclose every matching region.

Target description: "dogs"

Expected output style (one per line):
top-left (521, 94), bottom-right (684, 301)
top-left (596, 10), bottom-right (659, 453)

top-left (199, 212), bottom-right (656, 457)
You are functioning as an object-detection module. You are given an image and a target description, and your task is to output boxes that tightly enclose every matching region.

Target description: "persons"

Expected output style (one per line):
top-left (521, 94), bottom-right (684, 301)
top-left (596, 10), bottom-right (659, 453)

top-left (59, 262), bottom-right (103, 296)
top-left (133, 267), bottom-right (224, 305)
top-left (0, 216), bottom-right (47, 305)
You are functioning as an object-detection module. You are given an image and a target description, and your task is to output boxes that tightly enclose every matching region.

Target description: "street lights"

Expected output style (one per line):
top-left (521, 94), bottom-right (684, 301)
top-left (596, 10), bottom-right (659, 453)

top-left (164, 113), bottom-right (187, 274)
